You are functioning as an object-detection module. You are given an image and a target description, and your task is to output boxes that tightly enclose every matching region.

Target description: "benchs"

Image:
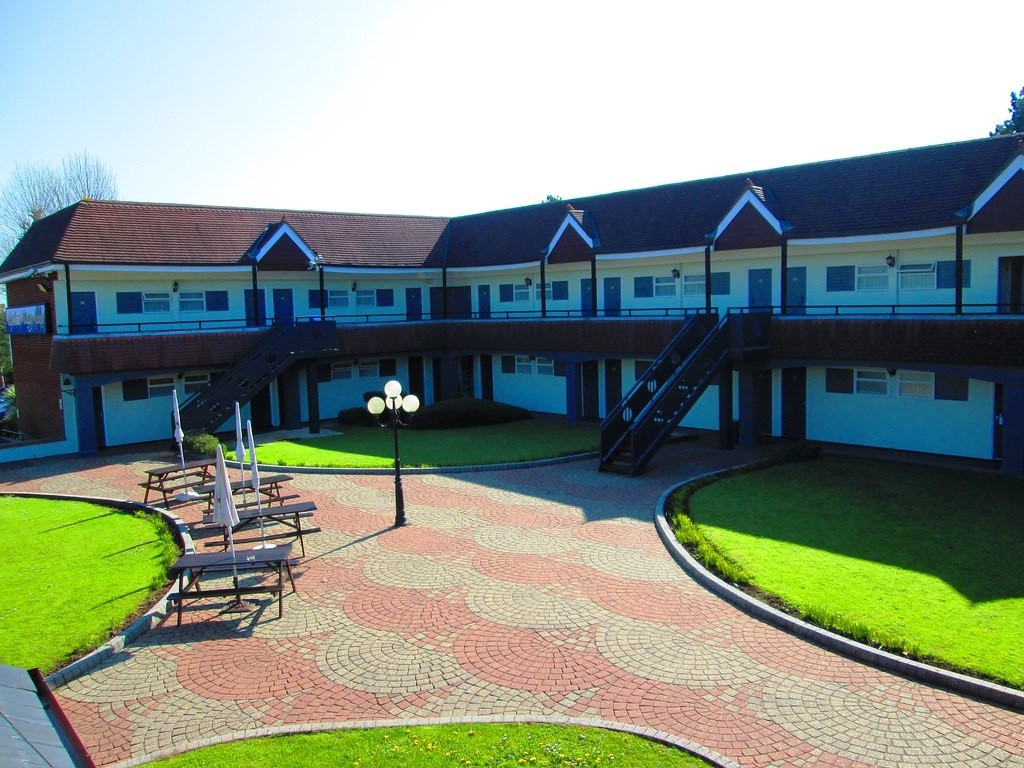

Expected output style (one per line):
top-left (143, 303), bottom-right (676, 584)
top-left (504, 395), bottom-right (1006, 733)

top-left (167, 585), bottom-right (284, 599)
top-left (197, 484), bottom-right (282, 500)
top-left (138, 470), bottom-right (203, 486)
top-left (193, 559), bottom-right (300, 572)
top-left (162, 476), bottom-right (230, 491)
top-left (202, 493), bottom-right (300, 514)
top-left (205, 512), bottom-right (313, 527)
top-left (205, 526), bottom-right (321, 547)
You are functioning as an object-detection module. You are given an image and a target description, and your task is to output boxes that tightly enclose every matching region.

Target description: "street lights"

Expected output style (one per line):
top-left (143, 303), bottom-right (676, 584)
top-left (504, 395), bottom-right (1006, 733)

top-left (367, 378), bottom-right (420, 527)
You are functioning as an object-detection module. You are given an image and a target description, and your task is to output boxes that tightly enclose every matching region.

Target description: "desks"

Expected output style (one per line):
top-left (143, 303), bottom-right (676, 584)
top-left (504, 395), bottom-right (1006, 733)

top-left (144, 458), bottom-right (216, 509)
top-left (173, 544), bottom-right (293, 627)
top-left (203, 500), bottom-right (317, 557)
top-left (192, 473), bottom-right (293, 516)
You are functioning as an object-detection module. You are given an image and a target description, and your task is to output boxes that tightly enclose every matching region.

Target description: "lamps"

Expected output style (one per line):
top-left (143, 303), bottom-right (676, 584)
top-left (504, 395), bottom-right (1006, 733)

top-left (37, 282), bottom-right (50, 293)
top-left (59, 378), bottom-right (75, 396)
top-left (529, 355), bottom-right (535, 360)
top-left (888, 367), bottom-right (896, 378)
top-left (886, 254), bottom-right (894, 269)
top-left (671, 267), bottom-right (680, 279)
top-left (173, 280), bottom-right (178, 293)
top-left (525, 277), bottom-right (531, 287)
top-left (352, 281), bottom-right (358, 291)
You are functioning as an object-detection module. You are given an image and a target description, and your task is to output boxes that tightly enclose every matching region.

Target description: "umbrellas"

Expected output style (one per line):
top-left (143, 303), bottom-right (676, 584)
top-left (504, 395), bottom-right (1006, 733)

top-left (212, 444), bottom-right (243, 603)
top-left (247, 420), bottom-right (266, 547)
top-left (173, 390), bottom-right (188, 495)
top-left (236, 402), bottom-right (248, 511)
